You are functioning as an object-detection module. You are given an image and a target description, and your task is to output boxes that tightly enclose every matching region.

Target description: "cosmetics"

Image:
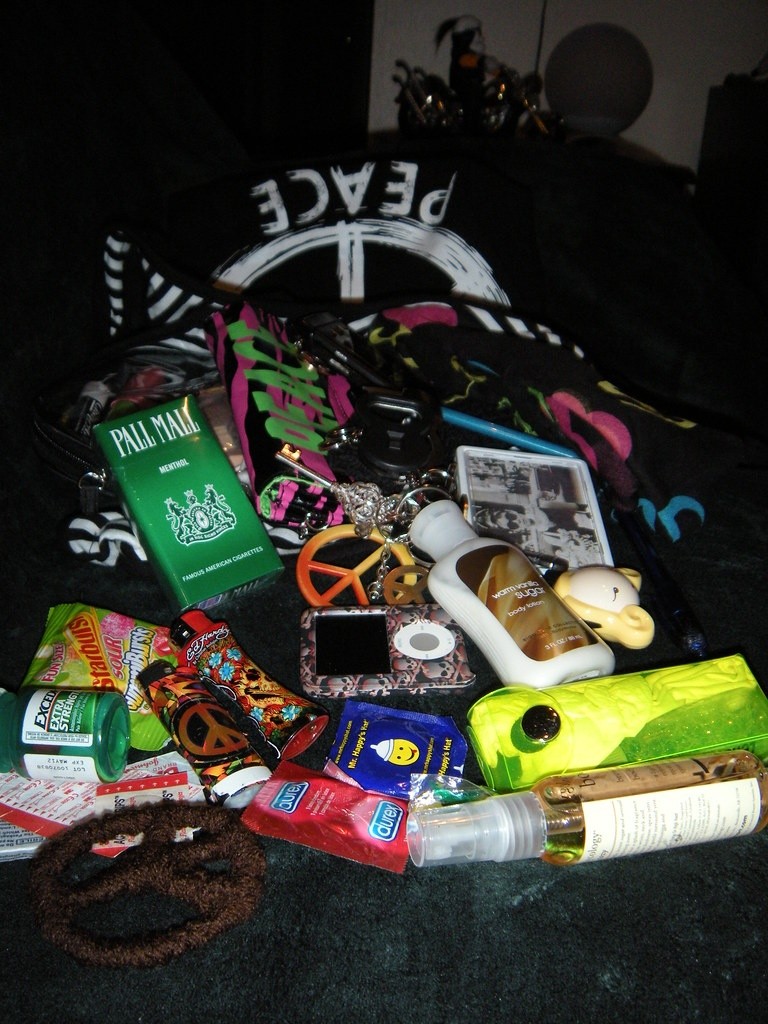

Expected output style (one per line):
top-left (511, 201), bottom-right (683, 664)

top-left (409, 500), bottom-right (615, 689)
top-left (407, 750), bottom-right (768, 868)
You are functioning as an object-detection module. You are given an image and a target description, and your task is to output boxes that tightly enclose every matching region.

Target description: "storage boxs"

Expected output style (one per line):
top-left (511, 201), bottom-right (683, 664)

top-left (93, 393), bottom-right (287, 619)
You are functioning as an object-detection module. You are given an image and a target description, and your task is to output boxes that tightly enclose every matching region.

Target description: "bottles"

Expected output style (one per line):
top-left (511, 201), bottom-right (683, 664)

top-left (168, 609), bottom-right (330, 761)
top-left (0, 686), bottom-right (131, 784)
top-left (405, 748), bottom-right (768, 868)
top-left (458, 651), bottom-right (768, 794)
top-left (409, 499), bottom-right (615, 689)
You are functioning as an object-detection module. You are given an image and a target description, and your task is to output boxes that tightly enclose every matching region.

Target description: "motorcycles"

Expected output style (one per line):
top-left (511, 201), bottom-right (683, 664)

top-left (397, 63), bottom-right (571, 155)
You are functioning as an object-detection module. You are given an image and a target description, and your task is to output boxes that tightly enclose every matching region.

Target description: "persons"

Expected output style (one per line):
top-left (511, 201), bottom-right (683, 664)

top-left (475, 500), bottom-right (597, 547)
